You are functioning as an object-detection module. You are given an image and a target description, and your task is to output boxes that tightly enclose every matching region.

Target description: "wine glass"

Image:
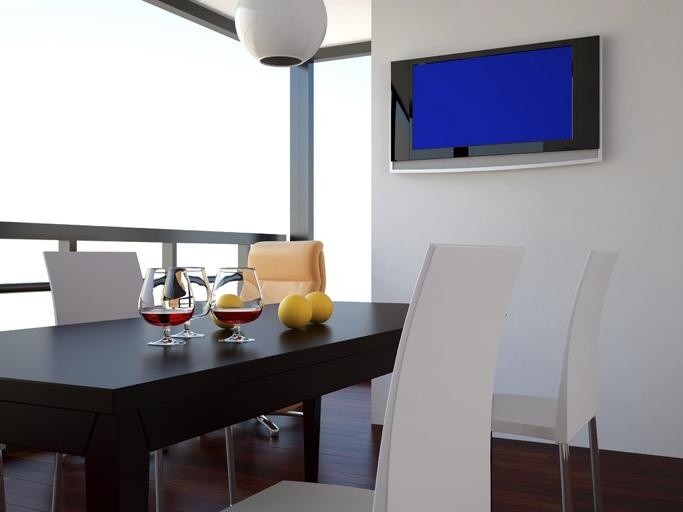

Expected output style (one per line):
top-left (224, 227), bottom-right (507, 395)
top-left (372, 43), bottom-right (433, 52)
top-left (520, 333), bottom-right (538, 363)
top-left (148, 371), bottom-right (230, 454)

top-left (137, 267), bottom-right (263, 347)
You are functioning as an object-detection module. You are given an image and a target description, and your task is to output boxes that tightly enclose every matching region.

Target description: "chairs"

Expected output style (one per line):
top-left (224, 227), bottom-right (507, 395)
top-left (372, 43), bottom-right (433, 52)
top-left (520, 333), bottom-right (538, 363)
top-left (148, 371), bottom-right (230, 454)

top-left (223, 241), bottom-right (534, 512)
top-left (239, 241), bottom-right (327, 438)
top-left (47, 251), bottom-right (243, 512)
top-left (491, 245), bottom-right (618, 512)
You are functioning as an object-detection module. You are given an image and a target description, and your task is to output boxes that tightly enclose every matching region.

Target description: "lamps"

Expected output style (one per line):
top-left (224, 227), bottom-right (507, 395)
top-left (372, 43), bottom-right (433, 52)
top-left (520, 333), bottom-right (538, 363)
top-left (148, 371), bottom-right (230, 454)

top-left (234, 0), bottom-right (328, 67)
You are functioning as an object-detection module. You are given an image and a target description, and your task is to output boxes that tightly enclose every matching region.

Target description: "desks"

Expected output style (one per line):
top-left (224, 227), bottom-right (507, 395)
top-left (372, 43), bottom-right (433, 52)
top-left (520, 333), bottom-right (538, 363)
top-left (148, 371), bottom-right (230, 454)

top-left (0, 302), bottom-right (409, 512)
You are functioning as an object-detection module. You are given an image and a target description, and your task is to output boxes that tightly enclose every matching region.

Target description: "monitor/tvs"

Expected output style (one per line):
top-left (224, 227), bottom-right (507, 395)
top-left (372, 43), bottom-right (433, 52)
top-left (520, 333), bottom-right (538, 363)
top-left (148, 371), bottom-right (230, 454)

top-left (390, 34), bottom-right (602, 175)
